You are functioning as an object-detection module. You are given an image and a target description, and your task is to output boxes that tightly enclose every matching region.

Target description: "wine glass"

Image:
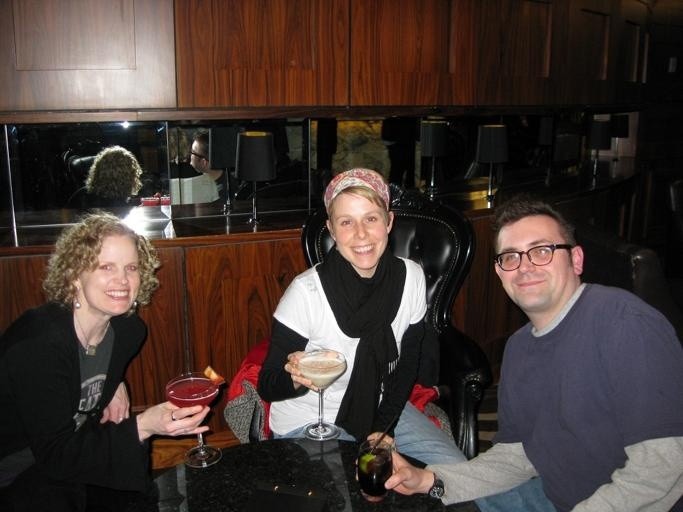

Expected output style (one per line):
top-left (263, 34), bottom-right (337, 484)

top-left (295, 349), bottom-right (347, 442)
top-left (164, 371), bottom-right (223, 469)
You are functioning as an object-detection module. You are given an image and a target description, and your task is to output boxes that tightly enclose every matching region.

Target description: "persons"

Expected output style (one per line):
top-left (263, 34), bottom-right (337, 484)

top-left (0, 212), bottom-right (211, 512)
top-left (190, 131), bottom-right (239, 208)
top-left (66, 145), bottom-right (142, 209)
top-left (148, 127), bottom-right (199, 195)
top-left (356, 194), bottom-right (683, 512)
top-left (258, 167), bottom-right (469, 465)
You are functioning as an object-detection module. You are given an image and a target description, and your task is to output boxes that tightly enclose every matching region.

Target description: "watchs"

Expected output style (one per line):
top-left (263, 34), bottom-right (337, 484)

top-left (424, 474), bottom-right (444, 502)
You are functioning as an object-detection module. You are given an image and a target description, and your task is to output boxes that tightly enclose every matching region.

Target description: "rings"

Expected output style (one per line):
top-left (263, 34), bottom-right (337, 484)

top-left (118, 418), bottom-right (123, 422)
top-left (171, 410), bottom-right (176, 421)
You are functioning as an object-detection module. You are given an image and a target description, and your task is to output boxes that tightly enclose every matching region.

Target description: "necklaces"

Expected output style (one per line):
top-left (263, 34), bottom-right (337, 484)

top-left (75, 310), bottom-right (108, 357)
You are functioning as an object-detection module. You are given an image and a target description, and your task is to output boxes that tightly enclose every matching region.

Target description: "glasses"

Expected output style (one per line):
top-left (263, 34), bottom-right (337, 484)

top-left (494, 244), bottom-right (571, 271)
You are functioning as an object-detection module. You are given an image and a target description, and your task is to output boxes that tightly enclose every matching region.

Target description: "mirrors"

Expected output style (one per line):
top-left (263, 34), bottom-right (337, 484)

top-left (2, 115), bottom-right (590, 229)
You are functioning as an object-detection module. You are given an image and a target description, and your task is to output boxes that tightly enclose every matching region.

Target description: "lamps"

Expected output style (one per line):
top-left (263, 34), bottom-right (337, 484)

top-left (477, 124), bottom-right (505, 201)
top-left (206, 126), bottom-right (245, 213)
top-left (418, 118), bottom-right (450, 188)
top-left (586, 121), bottom-right (607, 177)
top-left (236, 130), bottom-right (279, 226)
top-left (608, 112), bottom-right (629, 161)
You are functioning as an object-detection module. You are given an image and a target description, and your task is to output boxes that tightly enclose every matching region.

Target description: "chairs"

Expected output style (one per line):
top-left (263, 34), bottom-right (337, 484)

top-left (634, 249), bottom-right (683, 343)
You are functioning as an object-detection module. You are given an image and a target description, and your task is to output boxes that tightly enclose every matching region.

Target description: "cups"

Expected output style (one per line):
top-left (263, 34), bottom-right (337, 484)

top-left (357, 439), bottom-right (393, 496)
top-left (140, 197), bottom-right (171, 205)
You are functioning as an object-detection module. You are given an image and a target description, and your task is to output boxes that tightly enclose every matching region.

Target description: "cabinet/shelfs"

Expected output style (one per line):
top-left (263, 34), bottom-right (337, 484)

top-left (1, 0), bottom-right (657, 121)
top-left (0, 177), bottom-right (645, 473)
